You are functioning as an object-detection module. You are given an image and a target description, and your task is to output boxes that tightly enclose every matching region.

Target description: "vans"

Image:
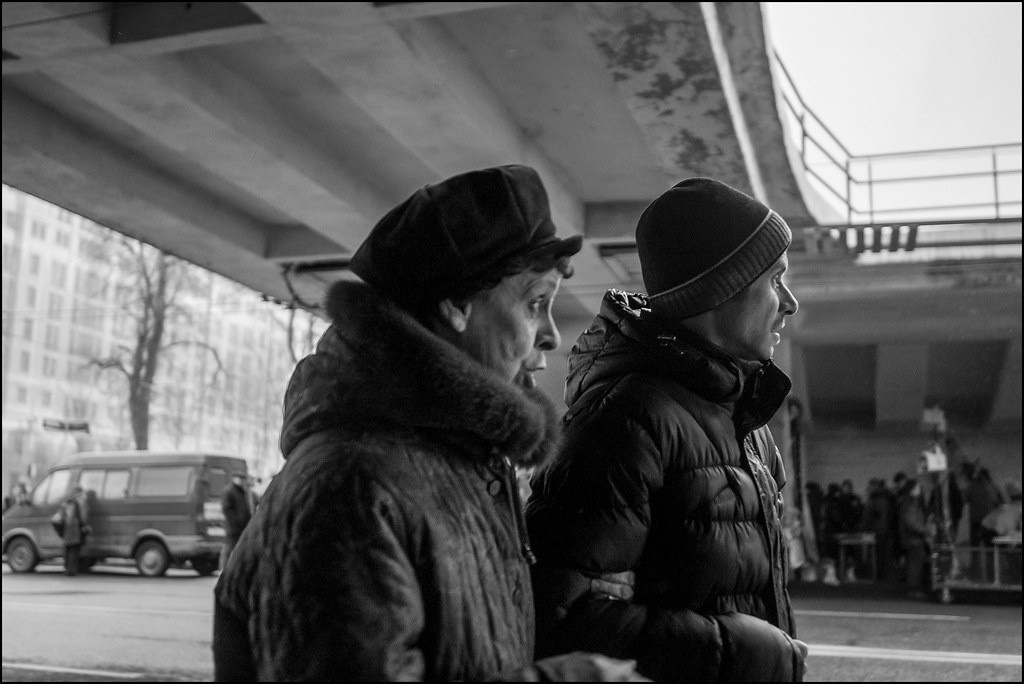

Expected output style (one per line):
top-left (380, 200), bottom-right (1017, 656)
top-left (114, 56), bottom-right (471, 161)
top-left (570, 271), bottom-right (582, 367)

top-left (2, 449), bottom-right (253, 578)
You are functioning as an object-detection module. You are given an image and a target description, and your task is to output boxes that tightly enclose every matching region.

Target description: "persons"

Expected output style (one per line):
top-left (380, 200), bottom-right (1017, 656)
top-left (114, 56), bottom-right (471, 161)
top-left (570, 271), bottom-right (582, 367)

top-left (213, 164), bottom-right (654, 682)
top-left (795, 459), bottom-right (1022, 603)
top-left (222, 472), bottom-right (253, 572)
top-left (51, 485), bottom-right (91, 574)
top-left (525, 177), bottom-right (810, 682)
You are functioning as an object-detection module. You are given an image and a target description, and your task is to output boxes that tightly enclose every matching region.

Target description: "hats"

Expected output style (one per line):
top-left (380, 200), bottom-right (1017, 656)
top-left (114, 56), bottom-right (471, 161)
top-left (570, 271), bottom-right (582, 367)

top-left (347, 164), bottom-right (582, 309)
top-left (230, 468), bottom-right (246, 478)
top-left (635, 177), bottom-right (792, 320)
